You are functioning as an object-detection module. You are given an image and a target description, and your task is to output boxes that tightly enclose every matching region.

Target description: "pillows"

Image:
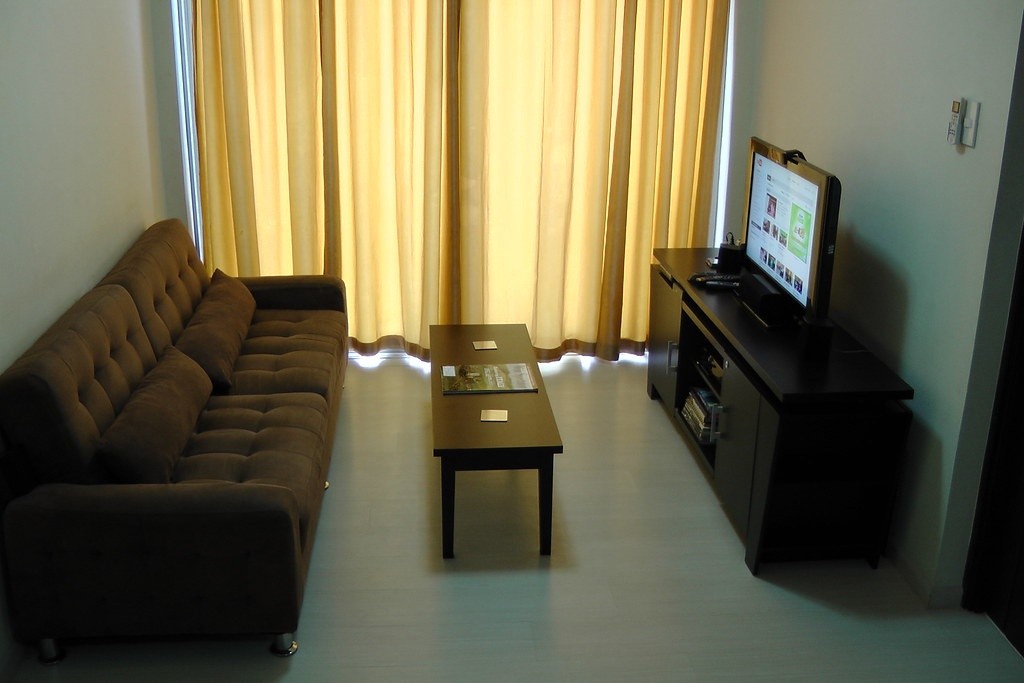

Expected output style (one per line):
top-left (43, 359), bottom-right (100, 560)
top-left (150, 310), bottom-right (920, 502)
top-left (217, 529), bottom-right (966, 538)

top-left (173, 268), bottom-right (256, 387)
top-left (96, 343), bottom-right (213, 482)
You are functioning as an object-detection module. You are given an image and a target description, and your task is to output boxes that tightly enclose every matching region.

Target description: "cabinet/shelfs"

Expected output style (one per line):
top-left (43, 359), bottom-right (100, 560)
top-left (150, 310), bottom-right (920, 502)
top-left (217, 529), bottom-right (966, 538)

top-left (647, 247), bottom-right (913, 576)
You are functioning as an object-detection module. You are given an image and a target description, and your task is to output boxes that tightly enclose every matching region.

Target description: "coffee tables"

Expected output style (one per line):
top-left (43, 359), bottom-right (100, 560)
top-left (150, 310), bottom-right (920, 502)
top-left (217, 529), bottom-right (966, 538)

top-left (430, 323), bottom-right (564, 561)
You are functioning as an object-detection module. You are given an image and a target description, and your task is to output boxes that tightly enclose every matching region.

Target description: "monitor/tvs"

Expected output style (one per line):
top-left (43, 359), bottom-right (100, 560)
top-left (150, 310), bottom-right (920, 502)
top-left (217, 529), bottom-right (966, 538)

top-left (734, 136), bottom-right (842, 330)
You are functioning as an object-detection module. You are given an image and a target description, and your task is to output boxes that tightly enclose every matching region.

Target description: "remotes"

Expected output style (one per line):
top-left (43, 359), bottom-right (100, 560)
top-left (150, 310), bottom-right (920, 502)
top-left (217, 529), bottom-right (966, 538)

top-left (687, 271), bottom-right (739, 289)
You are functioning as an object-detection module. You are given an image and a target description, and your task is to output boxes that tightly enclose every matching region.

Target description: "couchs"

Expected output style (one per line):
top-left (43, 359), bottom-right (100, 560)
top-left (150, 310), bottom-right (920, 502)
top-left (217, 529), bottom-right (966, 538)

top-left (1, 218), bottom-right (351, 662)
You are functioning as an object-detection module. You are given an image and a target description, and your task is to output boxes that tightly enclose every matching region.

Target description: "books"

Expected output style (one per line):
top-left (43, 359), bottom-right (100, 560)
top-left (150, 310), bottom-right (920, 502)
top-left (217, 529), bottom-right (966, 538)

top-left (681, 388), bottom-right (720, 440)
top-left (441, 363), bottom-right (538, 394)
